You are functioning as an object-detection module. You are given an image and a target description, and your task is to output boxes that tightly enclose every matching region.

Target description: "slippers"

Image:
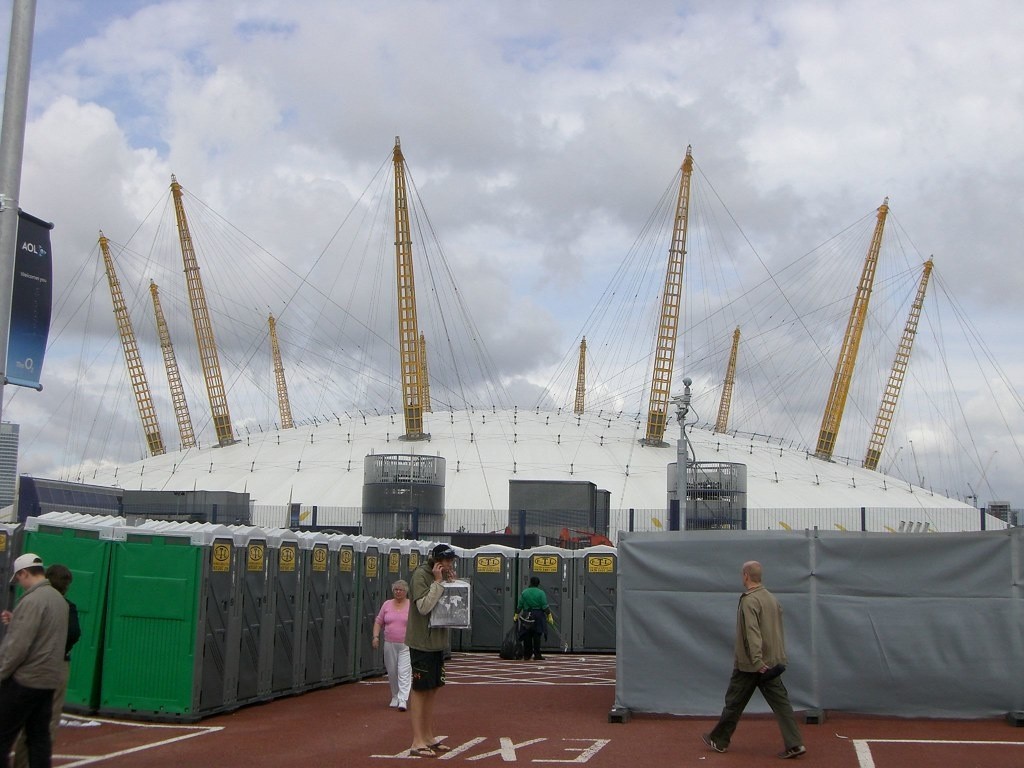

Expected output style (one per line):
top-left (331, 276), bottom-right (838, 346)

top-left (428, 742), bottom-right (450, 752)
top-left (408, 746), bottom-right (437, 757)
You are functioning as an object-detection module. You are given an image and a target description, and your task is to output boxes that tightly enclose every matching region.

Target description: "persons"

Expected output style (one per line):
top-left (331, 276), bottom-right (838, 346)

top-left (701, 561), bottom-right (806, 758)
top-left (372, 580), bottom-right (412, 710)
top-left (404, 544), bottom-right (456, 757)
top-left (514, 576), bottom-right (553, 660)
top-left (0, 554), bottom-right (81, 768)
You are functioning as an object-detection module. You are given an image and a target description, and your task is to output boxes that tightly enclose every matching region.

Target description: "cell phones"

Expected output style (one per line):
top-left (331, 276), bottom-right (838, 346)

top-left (428, 558), bottom-right (437, 569)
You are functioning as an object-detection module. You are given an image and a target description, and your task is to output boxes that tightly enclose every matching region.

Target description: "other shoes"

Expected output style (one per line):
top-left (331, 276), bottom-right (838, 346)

top-left (390, 698), bottom-right (398, 708)
top-left (398, 700), bottom-right (407, 712)
top-left (524, 656), bottom-right (529, 660)
top-left (534, 656), bottom-right (545, 660)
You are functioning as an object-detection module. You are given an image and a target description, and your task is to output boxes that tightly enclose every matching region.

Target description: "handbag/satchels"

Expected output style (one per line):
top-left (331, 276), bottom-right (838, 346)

top-left (428, 578), bottom-right (472, 630)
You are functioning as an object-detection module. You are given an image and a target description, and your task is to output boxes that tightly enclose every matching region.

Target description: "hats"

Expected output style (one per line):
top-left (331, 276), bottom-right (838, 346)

top-left (432, 544), bottom-right (459, 559)
top-left (9, 553), bottom-right (43, 583)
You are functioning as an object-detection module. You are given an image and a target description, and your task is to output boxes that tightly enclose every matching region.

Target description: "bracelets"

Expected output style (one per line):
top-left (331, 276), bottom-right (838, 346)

top-left (373, 636), bottom-right (379, 639)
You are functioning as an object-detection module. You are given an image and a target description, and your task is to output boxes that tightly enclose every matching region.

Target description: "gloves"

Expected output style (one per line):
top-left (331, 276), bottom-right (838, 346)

top-left (513, 613), bottom-right (519, 621)
top-left (547, 613), bottom-right (553, 625)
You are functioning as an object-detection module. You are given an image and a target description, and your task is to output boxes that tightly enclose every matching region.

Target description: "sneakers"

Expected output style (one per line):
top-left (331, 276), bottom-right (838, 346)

top-left (777, 746), bottom-right (806, 758)
top-left (701, 736), bottom-right (727, 753)
